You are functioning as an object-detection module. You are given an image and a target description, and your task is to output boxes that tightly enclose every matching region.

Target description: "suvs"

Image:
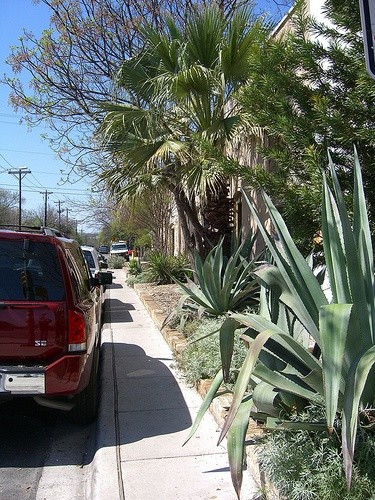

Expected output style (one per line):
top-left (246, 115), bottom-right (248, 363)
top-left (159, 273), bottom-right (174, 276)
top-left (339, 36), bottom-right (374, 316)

top-left (0, 223), bottom-right (103, 411)
top-left (110, 241), bottom-right (129, 261)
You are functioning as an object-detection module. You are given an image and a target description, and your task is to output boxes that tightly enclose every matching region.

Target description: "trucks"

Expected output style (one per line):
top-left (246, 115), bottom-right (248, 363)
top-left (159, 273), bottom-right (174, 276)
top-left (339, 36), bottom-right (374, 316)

top-left (99, 245), bottom-right (109, 254)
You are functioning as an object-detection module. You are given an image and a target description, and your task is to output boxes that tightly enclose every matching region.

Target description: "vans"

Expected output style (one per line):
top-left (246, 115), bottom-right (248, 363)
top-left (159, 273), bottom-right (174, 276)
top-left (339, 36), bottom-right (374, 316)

top-left (80, 246), bottom-right (106, 307)
